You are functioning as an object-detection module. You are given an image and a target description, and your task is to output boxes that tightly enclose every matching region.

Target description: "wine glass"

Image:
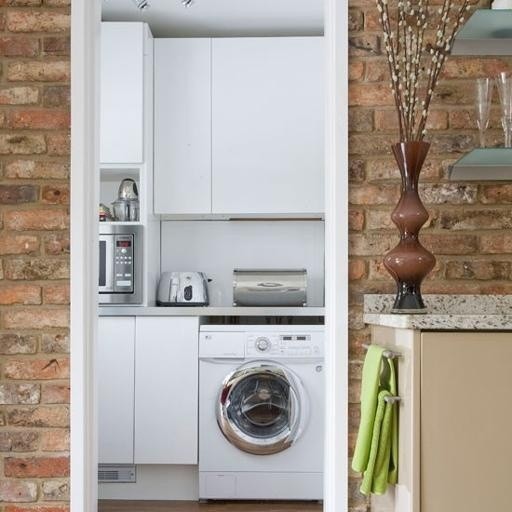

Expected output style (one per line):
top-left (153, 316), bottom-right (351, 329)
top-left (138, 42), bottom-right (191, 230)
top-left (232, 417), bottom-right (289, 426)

top-left (467, 69), bottom-right (512, 150)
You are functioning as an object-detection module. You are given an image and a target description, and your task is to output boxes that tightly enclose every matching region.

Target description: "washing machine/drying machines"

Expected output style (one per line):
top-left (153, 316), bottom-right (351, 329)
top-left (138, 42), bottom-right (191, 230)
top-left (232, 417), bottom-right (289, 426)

top-left (197, 322), bottom-right (325, 506)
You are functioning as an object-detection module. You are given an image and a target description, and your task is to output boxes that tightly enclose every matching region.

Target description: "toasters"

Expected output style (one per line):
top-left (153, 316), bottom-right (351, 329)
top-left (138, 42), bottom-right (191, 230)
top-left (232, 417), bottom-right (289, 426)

top-left (156, 270), bottom-right (213, 306)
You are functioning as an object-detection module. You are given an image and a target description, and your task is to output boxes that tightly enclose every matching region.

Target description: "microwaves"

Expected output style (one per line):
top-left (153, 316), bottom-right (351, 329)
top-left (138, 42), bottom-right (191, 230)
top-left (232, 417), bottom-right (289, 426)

top-left (97, 224), bottom-right (143, 304)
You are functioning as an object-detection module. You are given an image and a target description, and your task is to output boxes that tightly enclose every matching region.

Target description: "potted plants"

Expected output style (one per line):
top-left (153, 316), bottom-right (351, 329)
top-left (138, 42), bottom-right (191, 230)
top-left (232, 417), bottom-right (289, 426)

top-left (375, 0), bottom-right (478, 315)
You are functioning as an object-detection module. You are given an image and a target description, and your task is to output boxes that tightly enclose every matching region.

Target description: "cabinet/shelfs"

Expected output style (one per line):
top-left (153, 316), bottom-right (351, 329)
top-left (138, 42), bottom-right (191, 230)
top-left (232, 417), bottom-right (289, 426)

top-left (97, 316), bottom-right (199, 466)
top-left (101, 22), bottom-right (145, 166)
top-left (449, 7), bottom-right (512, 181)
top-left (154, 35), bottom-right (326, 216)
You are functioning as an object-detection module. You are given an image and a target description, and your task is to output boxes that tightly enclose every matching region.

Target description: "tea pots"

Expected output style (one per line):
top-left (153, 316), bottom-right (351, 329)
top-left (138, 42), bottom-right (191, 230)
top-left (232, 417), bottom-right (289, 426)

top-left (112, 179), bottom-right (139, 222)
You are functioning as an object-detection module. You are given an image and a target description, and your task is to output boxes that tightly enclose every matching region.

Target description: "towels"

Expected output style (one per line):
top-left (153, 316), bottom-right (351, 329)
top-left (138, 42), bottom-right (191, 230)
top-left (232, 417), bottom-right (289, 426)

top-left (352, 343), bottom-right (397, 497)
